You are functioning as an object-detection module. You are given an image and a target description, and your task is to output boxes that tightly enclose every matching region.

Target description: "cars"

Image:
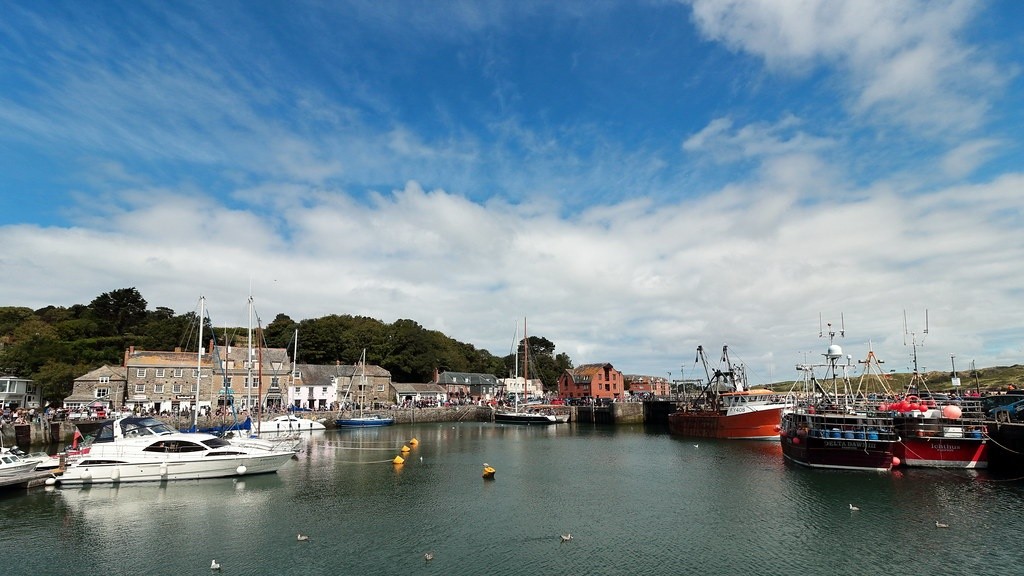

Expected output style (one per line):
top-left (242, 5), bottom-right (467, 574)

top-left (988, 398), bottom-right (1024, 421)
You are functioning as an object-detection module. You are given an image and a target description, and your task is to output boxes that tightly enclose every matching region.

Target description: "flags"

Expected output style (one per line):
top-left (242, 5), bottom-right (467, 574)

top-left (73, 429), bottom-right (80, 449)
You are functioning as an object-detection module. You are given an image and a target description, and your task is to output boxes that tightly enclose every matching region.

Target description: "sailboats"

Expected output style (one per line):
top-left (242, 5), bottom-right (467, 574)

top-left (1, 296), bottom-right (296, 484)
top-left (333, 346), bottom-right (395, 426)
top-left (252, 325), bottom-right (327, 431)
top-left (489, 317), bottom-right (572, 425)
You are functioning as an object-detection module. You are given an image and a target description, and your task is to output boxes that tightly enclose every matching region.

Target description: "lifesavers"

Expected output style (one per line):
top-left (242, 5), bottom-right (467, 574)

top-left (907, 396), bottom-right (920, 404)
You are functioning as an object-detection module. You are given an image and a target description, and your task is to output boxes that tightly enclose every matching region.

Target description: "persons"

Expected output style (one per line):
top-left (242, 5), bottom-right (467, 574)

top-left (950, 383), bottom-right (1020, 401)
top-left (0, 404), bottom-right (265, 424)
top-left (271, 394), bottom-right (603, 420)
top-left (868, 391), bottom-right (907, 401)
top-left (614, 392), bottom-right (815, 408)
top-left (929, 393), bottom-right (936, 409)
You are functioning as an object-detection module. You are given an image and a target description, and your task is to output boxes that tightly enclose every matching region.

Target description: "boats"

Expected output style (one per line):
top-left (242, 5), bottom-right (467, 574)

top-left (780, 309), bottom-right (989, 472)
top-left (663, 342), bottom-right (794, 442)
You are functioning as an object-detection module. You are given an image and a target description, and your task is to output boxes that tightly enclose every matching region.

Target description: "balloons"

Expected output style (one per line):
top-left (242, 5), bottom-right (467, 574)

top-left (780, 431), bottom-right (786, 436)
top-left (890, 403), bottom-right (928, 413)
top-left (893, 457), bottom-right (900, 465)
top-left (773, 425), bottom-right (781, 432)
top-left (797, 428), bottom-right (804, 435)
top-left (944, 405), bottom-right (962, 418)
top-left (810, 406), bottom-right (815, 413)
top-left (793, 438), bottom-right (800, 443)
